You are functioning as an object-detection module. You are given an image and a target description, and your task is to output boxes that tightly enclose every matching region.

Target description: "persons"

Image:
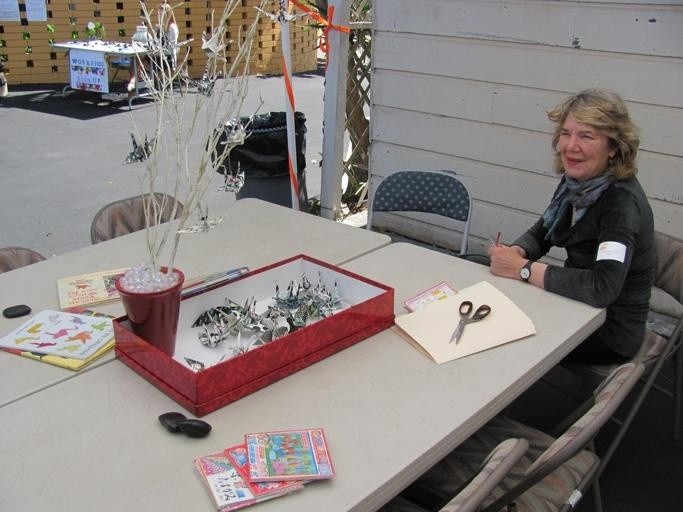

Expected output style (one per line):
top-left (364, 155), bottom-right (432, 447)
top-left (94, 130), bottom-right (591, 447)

top-left (124, 3), bottom-right (180, 93)
top-left (485, 85), bottom-right (659, 366)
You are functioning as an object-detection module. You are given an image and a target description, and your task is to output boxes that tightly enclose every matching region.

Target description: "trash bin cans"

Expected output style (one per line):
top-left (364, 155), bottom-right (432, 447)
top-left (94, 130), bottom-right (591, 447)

top-left (205, 111), bottom-right (308, 211)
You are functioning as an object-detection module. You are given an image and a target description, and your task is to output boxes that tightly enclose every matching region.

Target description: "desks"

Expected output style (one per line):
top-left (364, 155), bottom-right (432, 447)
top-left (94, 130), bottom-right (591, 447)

top-left (50, 40), bottom-right (179, 110)
top-left (0, 198), bottom-right (607, 512)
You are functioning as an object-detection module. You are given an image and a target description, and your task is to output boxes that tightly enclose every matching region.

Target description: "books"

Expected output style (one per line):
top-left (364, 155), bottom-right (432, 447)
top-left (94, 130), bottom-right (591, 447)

top-left (55, 266), bottom-right (131, 310)
top-left (389, 280), bottom-right (536, 365)
top-left (0, 304), bottom-right (118, 371)
top-left (192, 451), bottom-right (305, 512)
top-left (0, 309), bottom-right (117, 361)
top-left (224, 444), bottom-right (311, 495)
top-left (243, 427), bottom-right (333, 484)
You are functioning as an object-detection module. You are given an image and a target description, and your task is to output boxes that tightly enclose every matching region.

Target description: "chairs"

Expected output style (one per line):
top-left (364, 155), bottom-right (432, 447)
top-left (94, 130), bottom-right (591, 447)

top-left (410, 359), bottom-right (645, 511)
top-left (599, 229), bottom-right (682, 471)
top-left (366, 170), bottom-right (472, 257)
top-left (111, 57), bottom-right (131, 92)
top-left (91, 192), bottom-right (190, 244)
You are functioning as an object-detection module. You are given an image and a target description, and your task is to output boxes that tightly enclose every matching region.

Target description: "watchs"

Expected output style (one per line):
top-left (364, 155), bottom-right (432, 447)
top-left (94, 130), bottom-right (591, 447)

top-left (519, 259), bottom-right (538, 282)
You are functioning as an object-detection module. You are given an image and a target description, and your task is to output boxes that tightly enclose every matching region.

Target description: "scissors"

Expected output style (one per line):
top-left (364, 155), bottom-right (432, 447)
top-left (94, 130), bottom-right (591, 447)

top-left (449, 301), bottom-right (491, 345)
